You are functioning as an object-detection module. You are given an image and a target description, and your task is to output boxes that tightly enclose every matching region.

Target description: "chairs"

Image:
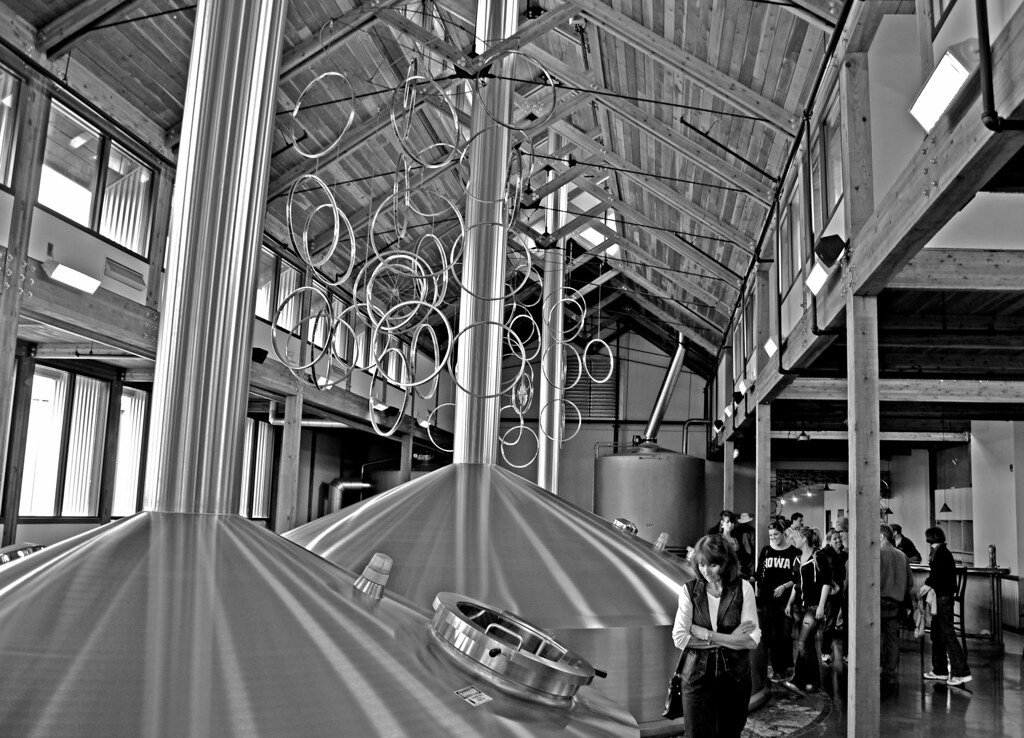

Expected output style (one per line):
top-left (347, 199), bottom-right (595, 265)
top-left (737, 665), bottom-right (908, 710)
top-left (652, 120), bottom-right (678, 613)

top-left (918, 566), bottom-right (969, 659)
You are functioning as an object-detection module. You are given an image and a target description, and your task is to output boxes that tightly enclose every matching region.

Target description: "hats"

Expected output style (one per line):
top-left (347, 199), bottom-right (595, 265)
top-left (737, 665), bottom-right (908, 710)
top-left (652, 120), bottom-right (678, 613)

top-left (737, 513), bottom-right (753, 523)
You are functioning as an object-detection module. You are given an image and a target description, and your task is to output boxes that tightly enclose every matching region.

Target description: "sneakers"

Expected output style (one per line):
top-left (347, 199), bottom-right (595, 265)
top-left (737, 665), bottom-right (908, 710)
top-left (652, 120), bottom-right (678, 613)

top-left (805, 684), bottom-right (815, 693)
top-left (821, 653), bottom-right (833, 662)
top-left (923, 671), bottom-right (948, 681)
top-left (946, 674), bottom-right (972, 686)
top-left (880, 667), bottom-right (896, 684)
top-left (782, 681), bottom-right (807, 697)
top-left (841, 655), bottom-right (849, 666)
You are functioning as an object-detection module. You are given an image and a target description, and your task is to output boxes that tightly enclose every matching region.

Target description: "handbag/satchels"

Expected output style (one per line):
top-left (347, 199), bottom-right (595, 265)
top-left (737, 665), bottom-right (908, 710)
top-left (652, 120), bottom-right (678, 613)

top-left (661, 677), bottom-right (684, 720)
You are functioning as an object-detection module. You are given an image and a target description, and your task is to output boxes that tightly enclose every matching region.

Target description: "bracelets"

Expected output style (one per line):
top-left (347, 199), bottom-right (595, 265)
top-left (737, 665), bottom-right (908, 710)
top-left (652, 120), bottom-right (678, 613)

top-left (705, 629), bottom-right (712, 644)
top-left (782, 584), bottom-right (787, 590)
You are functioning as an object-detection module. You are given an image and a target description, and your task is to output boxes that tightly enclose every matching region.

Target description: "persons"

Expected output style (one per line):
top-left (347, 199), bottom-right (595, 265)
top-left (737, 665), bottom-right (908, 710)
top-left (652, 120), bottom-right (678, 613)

top-left (756, 522), bottom-right (800, 682)
top-left (731, 512), bottom-right (756, 589)
top-left (917, 527), bottom-right (974, 686)
top-left (890, 523), bottom-right (923, 631)
top-left (672, 535), bottom-right (762, 738)
top-left (821, 516), bottom-right (848, 664)
top-left (875, 522), bottom-right (915, 692)
top-left (719, 510), bottom-right (752, 579)
top-left (785, 526), bottom-right (832, 694)
